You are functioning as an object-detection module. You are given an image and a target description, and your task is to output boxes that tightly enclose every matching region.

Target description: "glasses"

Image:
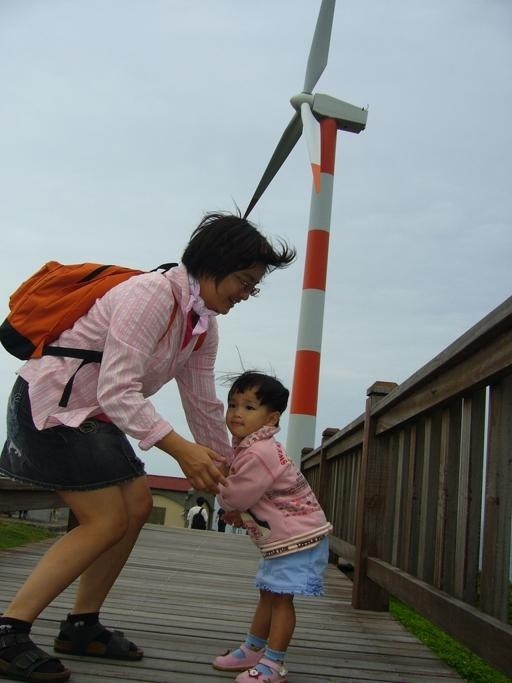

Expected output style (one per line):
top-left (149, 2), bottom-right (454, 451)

top-left (234, 279), bottom-right (262, 301)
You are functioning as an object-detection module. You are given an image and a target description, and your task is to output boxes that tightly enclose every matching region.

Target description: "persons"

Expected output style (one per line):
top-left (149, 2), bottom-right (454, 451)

top-left (0, 210), bottom-right (298, 683)
top-left (209, 344), bottom-right (337, 683)
top-left (186, 495), bottom-right (209, 531)
top-left (215, 506), bottom-right (228, 532)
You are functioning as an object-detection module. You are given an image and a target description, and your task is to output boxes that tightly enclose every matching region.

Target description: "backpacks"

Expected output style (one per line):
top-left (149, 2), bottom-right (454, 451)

top-left (191, 507), bottom-right (206, 529)
top-left (0, 261), bottom-right (178, 377)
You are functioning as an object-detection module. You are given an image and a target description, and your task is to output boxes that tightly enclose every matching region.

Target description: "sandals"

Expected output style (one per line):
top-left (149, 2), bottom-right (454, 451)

top-left (53, 613), bottom-right (144, 659)
top-left (0, 615), bottom-right (70, 681)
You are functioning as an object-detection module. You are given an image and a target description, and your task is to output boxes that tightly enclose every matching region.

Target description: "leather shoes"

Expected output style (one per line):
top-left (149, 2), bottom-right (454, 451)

top-left (212, 644), bottom-right (266, 670)
top-left (235, 659), bottom-right (288, 683)
